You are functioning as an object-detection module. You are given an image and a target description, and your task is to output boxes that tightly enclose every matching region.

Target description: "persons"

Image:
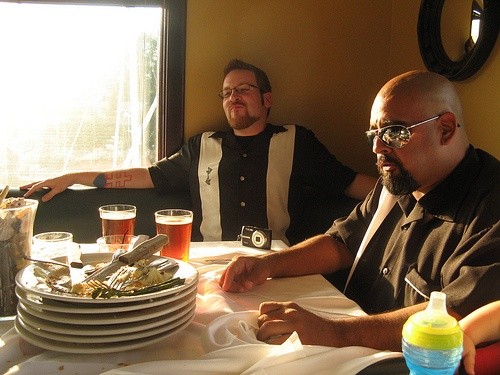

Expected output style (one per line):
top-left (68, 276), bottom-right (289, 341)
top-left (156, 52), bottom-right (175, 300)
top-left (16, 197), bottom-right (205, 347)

top-left (458, 300), bottom-right (500, 375)
top-left (464, 36), bottom-right (475, 56)
top-left (19, 58), bottom-right (377, 248)
top-left (218, 68), bottom-right (500, 353)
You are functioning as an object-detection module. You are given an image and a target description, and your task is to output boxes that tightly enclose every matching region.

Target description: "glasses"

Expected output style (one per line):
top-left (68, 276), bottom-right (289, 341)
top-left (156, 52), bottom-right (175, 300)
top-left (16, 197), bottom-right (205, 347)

top-left (218, 82), bottom-right (265, 98)
top-left (365, 112), bottom-right (460, 148)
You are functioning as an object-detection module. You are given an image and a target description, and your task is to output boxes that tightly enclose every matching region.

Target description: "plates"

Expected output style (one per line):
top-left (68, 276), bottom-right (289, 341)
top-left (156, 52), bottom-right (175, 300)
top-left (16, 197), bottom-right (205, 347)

top-left (13, 251), bottom-right (200, 354)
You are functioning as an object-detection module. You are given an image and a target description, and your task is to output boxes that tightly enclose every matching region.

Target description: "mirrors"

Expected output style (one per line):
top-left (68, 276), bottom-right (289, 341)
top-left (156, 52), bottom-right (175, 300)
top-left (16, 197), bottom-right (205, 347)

top-left (416, 0), bottom-right (500, 80)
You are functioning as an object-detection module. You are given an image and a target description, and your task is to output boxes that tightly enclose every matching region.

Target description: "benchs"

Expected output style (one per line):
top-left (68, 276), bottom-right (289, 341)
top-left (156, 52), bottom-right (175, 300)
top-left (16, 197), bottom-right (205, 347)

top-left (0, 189), bottom-right (377, 301)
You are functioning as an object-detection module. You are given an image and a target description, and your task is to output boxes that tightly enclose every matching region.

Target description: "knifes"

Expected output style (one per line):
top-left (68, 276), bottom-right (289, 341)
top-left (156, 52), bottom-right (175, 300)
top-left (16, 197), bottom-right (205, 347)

top-left (69, 234), bottom-right (169, 295)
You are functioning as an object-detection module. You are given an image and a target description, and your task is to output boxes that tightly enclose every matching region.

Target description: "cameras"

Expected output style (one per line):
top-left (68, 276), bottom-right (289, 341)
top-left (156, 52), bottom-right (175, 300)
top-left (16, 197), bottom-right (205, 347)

top-left (241, 225), bottom-right (271, 249)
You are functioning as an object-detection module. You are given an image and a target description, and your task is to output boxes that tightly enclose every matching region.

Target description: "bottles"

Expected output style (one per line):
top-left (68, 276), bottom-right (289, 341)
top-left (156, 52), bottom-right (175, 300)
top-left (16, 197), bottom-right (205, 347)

top-left (402, 291), bottom-right (464, 375)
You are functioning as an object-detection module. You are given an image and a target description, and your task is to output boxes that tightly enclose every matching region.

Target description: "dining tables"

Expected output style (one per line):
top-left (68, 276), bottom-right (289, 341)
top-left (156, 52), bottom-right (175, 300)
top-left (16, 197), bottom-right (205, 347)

top-left (0, 238), bottom-right (395, 375)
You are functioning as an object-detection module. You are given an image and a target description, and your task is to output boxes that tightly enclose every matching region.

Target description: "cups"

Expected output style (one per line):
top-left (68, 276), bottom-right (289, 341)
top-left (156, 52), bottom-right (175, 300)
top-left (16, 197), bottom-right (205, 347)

top-left (0, 197), bottom-right (39, 321)
top-left (155, 209), bottom-right (193, 263)
top-left (96, 235), bottom-right (138, 253)
top-left (99, 203), bottom-right (136, 252)
top-left (33, 231), bottom-right (73, 262)
